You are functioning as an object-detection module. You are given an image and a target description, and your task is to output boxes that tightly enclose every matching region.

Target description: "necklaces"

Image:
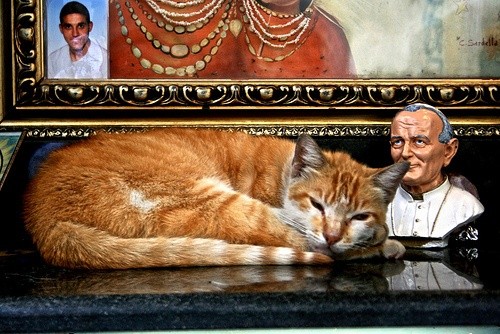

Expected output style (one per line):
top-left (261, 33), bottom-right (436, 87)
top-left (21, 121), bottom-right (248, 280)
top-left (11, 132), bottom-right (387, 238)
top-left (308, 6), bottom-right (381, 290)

top-left (389, 184), bottom-right (454, 236)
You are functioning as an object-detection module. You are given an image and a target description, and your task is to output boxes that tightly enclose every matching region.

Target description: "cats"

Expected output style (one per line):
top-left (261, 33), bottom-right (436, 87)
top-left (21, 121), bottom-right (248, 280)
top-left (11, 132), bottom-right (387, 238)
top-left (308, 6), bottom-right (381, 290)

top-left (24, 123), bottom-right (412, 267)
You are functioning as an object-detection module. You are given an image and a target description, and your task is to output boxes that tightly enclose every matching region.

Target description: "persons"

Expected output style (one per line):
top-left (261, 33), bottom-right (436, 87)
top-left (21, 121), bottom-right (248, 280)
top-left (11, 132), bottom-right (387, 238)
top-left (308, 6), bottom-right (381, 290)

top-left (385, 103), bottom-right (484, 238)
top-left (48, 1), bottom-right (108, 79)
